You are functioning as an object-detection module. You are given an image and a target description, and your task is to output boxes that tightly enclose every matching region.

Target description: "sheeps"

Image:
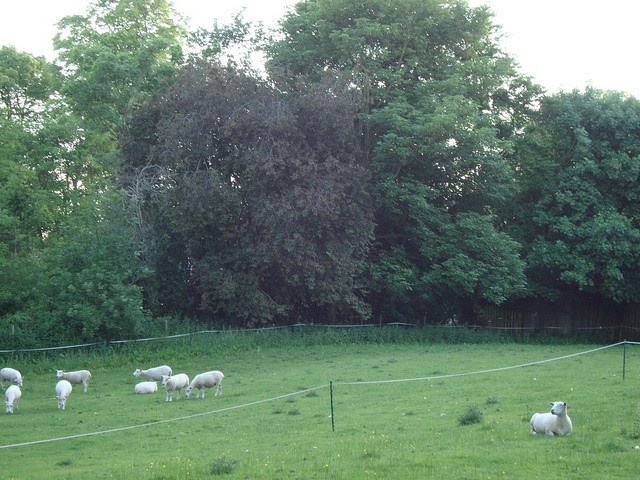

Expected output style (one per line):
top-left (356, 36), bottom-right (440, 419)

top-left (55, 380), bottom-right (72, 410)
top-left (186, 371), bottom-right (225, 399)
top-left (4, 385), bottom-right (22, 414)
top-left (530, 401), bottom-right (573, 436)
top-left (56, 369), bottom-right (92, 392)
top-left (161, 373), bottom-right (189, 401)
top-left (134, 381), bottom-right (158, 394)
top-left (133, 365), bottom-right (172, 382)
top-left (0, 367), bottom-right (23, 390)
